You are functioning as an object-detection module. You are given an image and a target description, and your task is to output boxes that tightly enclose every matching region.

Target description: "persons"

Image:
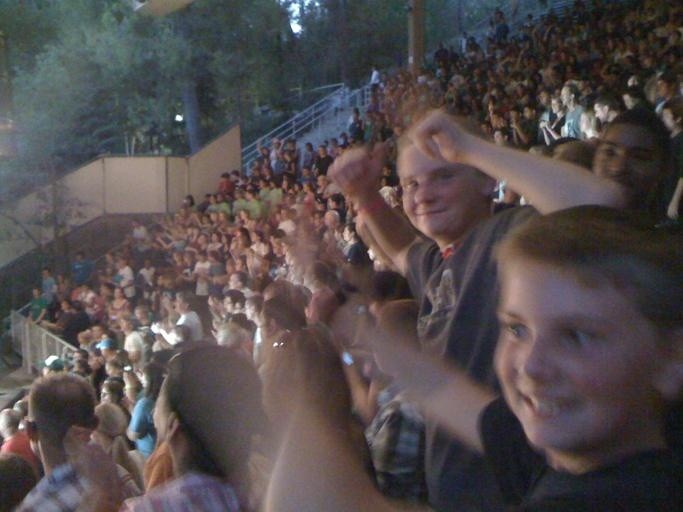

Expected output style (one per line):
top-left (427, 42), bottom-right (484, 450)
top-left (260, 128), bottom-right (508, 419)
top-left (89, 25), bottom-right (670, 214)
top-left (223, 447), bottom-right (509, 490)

top-left (0, 0), bottom-right (683, 511)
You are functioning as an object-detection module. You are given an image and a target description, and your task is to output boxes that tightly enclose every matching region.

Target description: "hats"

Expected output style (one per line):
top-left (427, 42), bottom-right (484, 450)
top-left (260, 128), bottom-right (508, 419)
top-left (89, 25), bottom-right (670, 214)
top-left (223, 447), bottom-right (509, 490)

top-left (33, 355), bottom-right (64, 371)
top-left (95, 339), bottom-right (113, 350)
